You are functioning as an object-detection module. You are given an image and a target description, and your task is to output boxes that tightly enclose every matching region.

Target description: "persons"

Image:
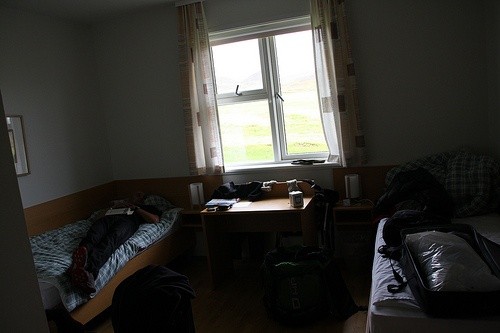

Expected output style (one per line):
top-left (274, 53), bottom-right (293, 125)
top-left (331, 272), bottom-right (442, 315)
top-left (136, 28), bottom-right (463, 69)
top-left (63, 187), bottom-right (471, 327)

top-left (65, 190), bottom-right (161, 293)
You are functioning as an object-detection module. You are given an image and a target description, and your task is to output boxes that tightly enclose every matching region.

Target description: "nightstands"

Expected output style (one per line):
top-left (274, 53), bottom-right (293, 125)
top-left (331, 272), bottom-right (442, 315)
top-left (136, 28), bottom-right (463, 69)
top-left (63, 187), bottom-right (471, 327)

top-left (178, 204), bottom-right (203, 234)
top-left (332, 200), bottom-right (376, 237)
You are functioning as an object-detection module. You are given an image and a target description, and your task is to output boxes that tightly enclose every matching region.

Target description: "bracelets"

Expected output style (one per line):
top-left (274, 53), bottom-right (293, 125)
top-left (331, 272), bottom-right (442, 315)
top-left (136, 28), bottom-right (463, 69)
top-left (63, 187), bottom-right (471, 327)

top-left (133, 206), bottom-right (140, 210)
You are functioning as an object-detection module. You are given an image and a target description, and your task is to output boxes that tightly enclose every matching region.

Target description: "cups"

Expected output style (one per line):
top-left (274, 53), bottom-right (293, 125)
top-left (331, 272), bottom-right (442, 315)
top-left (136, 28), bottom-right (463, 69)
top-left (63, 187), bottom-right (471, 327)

top-left (288, 190), bottom-right (303, 207)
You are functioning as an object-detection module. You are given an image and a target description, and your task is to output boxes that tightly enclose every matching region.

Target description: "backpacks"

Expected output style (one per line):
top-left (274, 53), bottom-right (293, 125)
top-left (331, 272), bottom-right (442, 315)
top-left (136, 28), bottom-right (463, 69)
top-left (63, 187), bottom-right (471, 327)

top-left (382, 210), bottom-right (451, 247)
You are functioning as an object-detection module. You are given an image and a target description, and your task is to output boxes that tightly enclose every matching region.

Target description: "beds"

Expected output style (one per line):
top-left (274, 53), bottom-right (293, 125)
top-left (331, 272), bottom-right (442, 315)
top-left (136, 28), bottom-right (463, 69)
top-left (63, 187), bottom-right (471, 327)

top-left (364, 204), bottom-right (500, 333)
top-left (30, 208), bottom-right (194, 333)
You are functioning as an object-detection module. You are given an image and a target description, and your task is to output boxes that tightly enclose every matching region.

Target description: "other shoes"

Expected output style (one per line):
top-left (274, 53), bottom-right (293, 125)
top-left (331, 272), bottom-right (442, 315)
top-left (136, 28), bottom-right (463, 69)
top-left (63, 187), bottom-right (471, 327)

top-left (68, 264), bottom-right (96, 294)
top-left (66, 246), bottom-right (88, 277)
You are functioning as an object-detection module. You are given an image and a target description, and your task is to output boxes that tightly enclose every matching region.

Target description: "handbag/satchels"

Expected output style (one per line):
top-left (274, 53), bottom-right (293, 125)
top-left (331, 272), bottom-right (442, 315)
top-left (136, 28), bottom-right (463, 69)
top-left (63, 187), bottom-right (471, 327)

top-left (261, 245), bottom-right (359, 324)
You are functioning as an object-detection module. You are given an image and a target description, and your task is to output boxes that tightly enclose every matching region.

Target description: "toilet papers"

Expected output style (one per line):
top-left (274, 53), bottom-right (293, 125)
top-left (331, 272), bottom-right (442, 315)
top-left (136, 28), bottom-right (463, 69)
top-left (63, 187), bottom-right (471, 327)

top-left (345, 174), bottom-right (360, 198)
top-left (189, 182), bottom-right (205, 205)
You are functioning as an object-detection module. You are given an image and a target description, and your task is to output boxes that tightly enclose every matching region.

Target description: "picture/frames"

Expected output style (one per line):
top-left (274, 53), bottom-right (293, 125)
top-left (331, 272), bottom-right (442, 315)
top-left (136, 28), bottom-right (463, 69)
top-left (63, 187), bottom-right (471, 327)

top-left (6, 114), bottom-right (32, 177)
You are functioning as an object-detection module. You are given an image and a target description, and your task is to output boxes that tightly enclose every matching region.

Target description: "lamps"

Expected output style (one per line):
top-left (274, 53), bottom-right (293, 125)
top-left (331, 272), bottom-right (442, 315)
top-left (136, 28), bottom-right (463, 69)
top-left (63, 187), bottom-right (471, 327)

top-left (344, 173), bottom-right (360, 204)
top-left (188, 182), bottom-right (204, 209)
top-left (199, 194), bottom-right (316, 290)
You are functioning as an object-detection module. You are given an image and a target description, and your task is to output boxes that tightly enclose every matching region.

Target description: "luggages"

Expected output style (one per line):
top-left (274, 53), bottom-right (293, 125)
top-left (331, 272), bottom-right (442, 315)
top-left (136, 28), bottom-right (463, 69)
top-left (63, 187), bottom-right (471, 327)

top-left (112, 265), bottom-right (195, 333)
top-left (390, 223), bottom-right (500, 317)
